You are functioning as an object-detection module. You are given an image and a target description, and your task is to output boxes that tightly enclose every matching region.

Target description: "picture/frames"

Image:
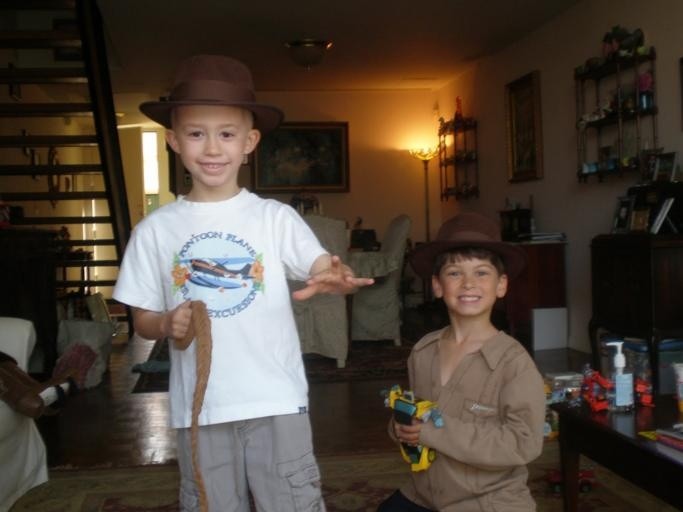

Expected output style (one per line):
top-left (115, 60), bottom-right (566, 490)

top-left (249, 121), bottom-right (351, 195)
top-left (8, 61), bottom-right (23, 101)
top-left (31, 148), bottom-right (42, 182)
top-left (502, 69), bottom-right (544, 184)
top-left (165, 139), bottom-right (255, 198)
top-left (649, 196), bottom-right (675, 235)
top-left (610, 194), bottom-right (637, 235)
top-left (652, 151), bottom-right (678, 183)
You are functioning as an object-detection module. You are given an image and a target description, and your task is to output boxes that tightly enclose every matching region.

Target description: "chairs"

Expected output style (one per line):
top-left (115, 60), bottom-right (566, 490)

top-left (289, 215), bottom-right (349, 369)
top-left (350, 212), bottom-right (413, 347)
top-left (0, 317), bottom-right (49, 512)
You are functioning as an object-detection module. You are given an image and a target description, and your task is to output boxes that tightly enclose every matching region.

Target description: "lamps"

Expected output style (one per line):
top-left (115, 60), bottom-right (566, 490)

top-left (281, 38), bottom-right (329, 73)
top-left (408, 140), bottom-right (446, 243)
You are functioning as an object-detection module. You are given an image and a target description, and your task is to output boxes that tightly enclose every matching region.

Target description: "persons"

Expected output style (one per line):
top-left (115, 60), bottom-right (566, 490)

top-left (377, 212), bottom-right (543, 512)
top-left (112, 56), bottom-right (372, 512)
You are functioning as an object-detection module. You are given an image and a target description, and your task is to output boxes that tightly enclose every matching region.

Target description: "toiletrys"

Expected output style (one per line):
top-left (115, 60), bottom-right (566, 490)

top-left (605, 340), bottom-right (636, 414)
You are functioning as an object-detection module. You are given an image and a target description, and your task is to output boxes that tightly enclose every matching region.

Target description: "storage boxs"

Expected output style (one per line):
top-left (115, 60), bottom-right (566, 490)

top-left (606, 337), bottom-right (683, 398)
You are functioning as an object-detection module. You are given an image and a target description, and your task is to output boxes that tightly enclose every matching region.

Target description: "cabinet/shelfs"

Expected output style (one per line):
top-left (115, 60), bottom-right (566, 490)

top-left (573, 44), bottom-right (658, 184)
top-left (587, 232), bottom-right (683, 399)
top-left (439, 116), bottom-right (480, 202)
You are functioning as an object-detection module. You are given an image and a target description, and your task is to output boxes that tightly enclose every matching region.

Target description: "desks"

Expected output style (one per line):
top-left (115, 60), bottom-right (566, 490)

top-left (406, 239), bottom-right (567, 339)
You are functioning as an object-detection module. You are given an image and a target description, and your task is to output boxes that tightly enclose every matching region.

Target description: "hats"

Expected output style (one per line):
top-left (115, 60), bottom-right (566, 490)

top-left (407, 212), bottom-right (529, 283)
top-left (137, 54), bottom-right (286, 134)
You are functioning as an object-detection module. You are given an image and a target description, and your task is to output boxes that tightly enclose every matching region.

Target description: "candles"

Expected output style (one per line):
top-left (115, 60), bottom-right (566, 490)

top-left (295, 199), bottom-right (323, 215)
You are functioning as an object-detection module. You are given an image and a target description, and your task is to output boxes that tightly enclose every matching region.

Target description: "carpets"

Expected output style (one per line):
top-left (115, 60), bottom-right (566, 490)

top-left (7, 438), bottom-right (683, 512)
top-left (130, 334), bottom-right (421, 384)
